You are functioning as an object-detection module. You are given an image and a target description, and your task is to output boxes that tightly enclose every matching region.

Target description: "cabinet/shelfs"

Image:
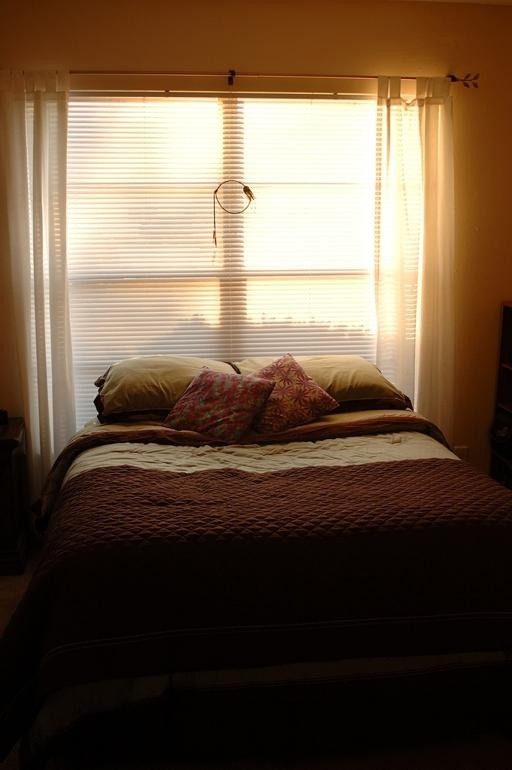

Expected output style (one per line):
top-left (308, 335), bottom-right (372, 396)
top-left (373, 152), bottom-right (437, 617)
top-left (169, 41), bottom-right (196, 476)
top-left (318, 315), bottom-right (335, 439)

top-left (487, 302), bottom-right (512, 491)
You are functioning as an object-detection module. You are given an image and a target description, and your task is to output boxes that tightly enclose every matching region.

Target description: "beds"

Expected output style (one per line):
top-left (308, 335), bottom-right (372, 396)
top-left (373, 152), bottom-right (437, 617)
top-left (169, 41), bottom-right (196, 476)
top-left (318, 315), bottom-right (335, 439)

top-left (36, 406), bottom-right (512, 765)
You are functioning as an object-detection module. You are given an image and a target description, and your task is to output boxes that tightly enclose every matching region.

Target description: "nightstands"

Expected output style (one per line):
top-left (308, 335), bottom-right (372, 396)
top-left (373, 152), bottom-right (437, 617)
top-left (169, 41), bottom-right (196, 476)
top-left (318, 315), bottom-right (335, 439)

top-left (0, 415), bottom-right (26, 576)
